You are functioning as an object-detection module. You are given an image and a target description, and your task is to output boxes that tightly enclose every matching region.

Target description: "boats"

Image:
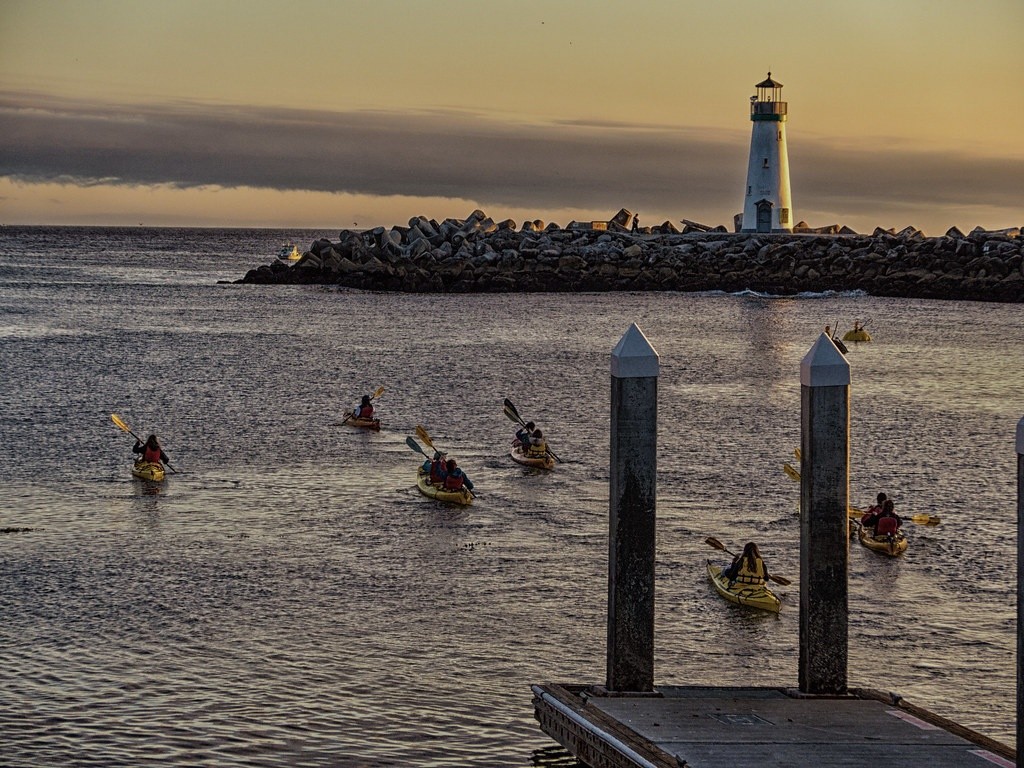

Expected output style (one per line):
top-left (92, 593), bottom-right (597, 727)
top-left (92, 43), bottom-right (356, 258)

top-left (834, 337), bottom-right (848, 354)
top-left (705, 558), bottom-right (780, 613)
top-left (278, 242), bottom-right (301, 259)
top-left (343, 408), bottom-right (380, 428)
top-left (511, 444), bottom-right (554, 469)
top-left (859, 521), bottom-right (907, 556)
top-left (844, 329), bottom-right (871, 341)
top-left (416, 463), bottom-right (472, 505)
top-left (133, 454), bottom-right (165, 480)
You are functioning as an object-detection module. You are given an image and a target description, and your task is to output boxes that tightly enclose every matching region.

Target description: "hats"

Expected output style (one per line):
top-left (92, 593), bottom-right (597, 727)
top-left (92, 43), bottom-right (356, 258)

top-left (433, 451), bottom-right (448, 460)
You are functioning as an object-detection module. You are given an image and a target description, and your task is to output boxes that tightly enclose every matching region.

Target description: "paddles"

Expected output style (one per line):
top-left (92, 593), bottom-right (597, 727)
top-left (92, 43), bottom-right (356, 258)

top-left (405, 434), bottom-right (471, 499)
top-left (111, 412), bottom-right (178, 475)
top-left (339, 388), bottom-right (388, 428)
top-left (848, 505), bottom-right (940, 526)
top-left (416, 424), bottom-right (477, 499)
top-left (831, 320), bottom-right (838, 340)
top-left (705, 534), bottom-right (792, 589)
top-left (784, 462), bottom-right (799, 486)
top-left (501, 395), bottom-right (561, 466)
top-left (792, 447), bottom-right (801, 461)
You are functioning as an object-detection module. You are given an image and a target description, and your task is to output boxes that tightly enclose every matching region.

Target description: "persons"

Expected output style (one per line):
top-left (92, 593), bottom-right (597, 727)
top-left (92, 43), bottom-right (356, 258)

top-left (725, 542), bottom-right (769, 590)
top-left (430, 451), bottom-right (474, 491)
top-left (515, 421), bottom-right (546, 458)
top-left (133, 435), bottom-right (169, 465)
top-left (825, 325), bottom-right (830, 336)
top-left (351, 395), bottom-right (373, 419)
top-left (861, 492), bottom-right (903, 537)
